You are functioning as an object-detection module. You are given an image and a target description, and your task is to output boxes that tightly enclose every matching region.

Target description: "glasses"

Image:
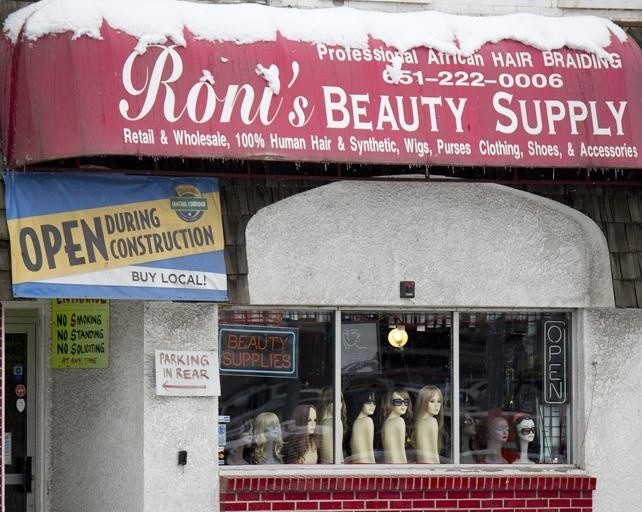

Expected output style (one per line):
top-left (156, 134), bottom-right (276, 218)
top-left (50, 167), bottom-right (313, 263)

top-left (522, 427), bottom-right (535, 435)
top-left (392, 398), bottom-right (409, 406)
top-left (365, 399), bottom-right (376, 404)
top-left (463, 419), bottom-right (474, 426)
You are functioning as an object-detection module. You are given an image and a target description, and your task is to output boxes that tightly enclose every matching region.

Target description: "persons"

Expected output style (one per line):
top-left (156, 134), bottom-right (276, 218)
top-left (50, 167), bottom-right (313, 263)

top-left (348, 388), bottom-right (384, 464)
top-left (317, 387), bottom-right (348, 463)
top-left (252, 412), bottom-right (289, 465)
top-left (480, 415), bottom-right (510, 465)
top-left (380, 385), bottom-right (415, 464)
top-left (458, 412), bottom-right (477, 464)
top-left (287, 404), bottom-right (322, 464)
top-left (411, 385), bottom-right (445, 464)
top-left (513, 414), bottom-right (536, 468)
top-left (225, 406), bottom-right (254, 464)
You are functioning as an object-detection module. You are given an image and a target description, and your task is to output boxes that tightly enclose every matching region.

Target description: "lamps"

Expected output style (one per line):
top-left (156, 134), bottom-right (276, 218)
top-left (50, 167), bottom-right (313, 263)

top-left (386, 328), bottom-right (410, 354)
